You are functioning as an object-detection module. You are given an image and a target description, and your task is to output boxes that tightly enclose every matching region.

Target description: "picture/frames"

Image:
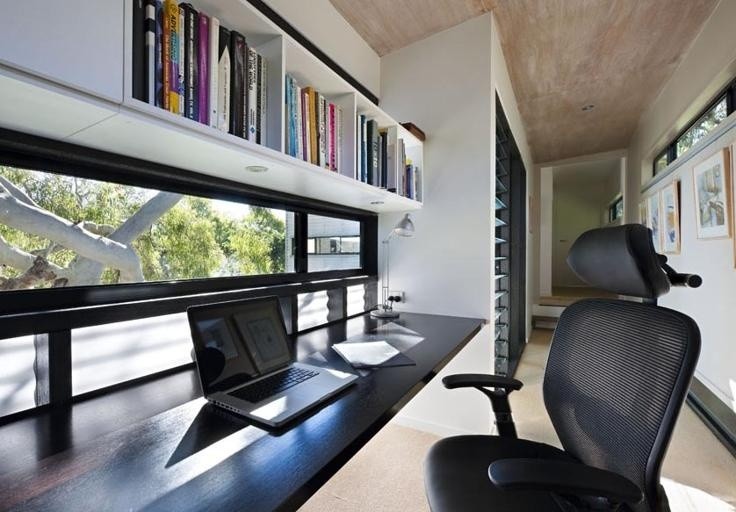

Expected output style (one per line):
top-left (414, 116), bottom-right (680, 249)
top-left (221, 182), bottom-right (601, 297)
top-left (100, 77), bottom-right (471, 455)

top-left (690, 145), bottom-right (734, 242)
top-left (635, 176), bottom-right (683, 256)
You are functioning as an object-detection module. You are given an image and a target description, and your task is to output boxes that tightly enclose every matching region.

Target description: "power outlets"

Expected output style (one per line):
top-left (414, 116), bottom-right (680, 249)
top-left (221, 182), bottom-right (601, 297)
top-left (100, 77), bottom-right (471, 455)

top-left (388, 291), bottom-right (404, 305)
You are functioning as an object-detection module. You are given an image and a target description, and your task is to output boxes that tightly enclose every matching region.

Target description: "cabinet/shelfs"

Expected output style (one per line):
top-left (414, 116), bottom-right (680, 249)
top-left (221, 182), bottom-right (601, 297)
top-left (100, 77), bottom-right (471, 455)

top-left (491, 86), bottom-right (529, 395)
top-left (0, 1), bottom-right (430, 213)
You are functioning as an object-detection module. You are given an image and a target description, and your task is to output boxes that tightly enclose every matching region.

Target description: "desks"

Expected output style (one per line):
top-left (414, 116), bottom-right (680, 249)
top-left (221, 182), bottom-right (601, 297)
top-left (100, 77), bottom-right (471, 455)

top-left (1, 304), bottom-right (489, 511)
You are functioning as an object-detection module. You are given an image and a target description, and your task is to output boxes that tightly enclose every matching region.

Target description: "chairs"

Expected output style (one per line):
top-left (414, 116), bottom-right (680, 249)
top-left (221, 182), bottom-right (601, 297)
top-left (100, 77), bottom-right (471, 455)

top-left (421, 222), bottom-right (704, 512)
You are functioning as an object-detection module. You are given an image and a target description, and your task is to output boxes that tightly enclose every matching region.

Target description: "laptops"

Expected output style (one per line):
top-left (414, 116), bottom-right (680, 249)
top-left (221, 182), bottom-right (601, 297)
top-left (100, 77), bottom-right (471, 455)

top-left (187, 295), bottom-right (359, 429)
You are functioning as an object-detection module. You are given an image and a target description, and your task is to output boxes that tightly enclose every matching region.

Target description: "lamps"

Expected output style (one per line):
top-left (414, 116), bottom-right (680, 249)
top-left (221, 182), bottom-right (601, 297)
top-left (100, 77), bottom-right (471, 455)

top-left (369, 211), bottom-right (417, 320)
